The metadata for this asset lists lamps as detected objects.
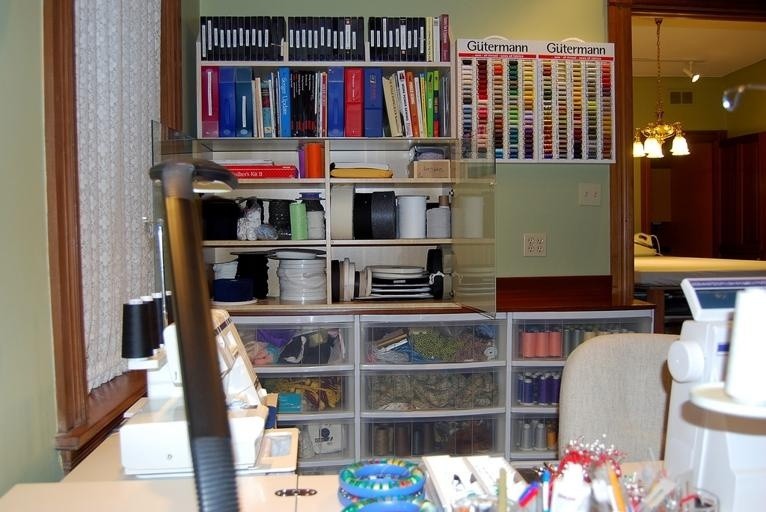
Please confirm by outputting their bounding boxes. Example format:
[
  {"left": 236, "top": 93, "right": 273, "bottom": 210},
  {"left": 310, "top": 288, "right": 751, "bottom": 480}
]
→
[
  {"left": 634, "top": 19, "right": 703, "bottom": 160},
  {"left": 147, "top": 158, "right": 242, "bottom": 508},
  {"left": 720, "top": 81, "right": 764, "bottom": 113}
]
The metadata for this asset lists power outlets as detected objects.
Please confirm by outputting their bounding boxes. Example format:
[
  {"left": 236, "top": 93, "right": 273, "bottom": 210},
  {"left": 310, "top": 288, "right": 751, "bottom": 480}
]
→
[{"left": 523, "top": 232, "right": 547, "bottom": 257}]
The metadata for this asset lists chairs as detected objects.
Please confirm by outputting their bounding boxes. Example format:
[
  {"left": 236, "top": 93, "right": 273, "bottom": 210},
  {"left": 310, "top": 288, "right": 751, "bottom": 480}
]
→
[{"left": 556, "top": 329, "right": 682, "bottom": 466}]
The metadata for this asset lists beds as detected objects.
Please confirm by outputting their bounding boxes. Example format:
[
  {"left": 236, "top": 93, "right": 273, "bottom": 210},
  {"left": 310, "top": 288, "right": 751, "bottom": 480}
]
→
[{"left": 634, "top": 232, "right": 766, "bottom": 334}]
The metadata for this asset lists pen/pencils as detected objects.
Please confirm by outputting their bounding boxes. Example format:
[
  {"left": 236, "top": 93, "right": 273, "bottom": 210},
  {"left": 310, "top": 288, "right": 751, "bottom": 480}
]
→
[
  {"left": 604, "top": 448, "right": 705, "bottom": 512},
  {"left": 510, "top": 470, "right": 550, "bottom": 512},
  {"left": 499, "top": 468, "right": 506, "bottom": 512}
]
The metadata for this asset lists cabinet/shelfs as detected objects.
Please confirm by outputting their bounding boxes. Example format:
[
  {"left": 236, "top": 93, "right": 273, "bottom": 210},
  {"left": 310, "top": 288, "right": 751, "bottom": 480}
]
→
[
  {"left": 194, "top": 27, "right": 458, "bottom": 141},
  {"left": 228, "top": 310, "right": 651, "bottom": 475},
  {"left": 639, "top": 127, "right": 765, "bottom": 260},
  {"left": 153, "top": 148, "right": 454, "bottom": 314}
]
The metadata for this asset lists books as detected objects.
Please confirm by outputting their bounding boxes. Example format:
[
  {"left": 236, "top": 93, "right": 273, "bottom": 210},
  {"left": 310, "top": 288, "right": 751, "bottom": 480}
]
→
[{"left": 200, "top": 14, "right": 451, "bottom": 136}]
